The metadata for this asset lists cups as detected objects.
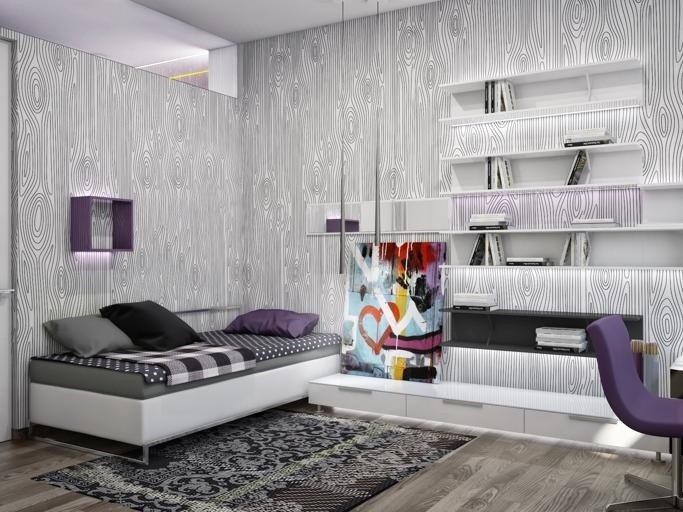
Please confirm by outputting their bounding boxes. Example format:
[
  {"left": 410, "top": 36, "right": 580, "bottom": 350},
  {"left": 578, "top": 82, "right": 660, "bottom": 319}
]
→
[{"left": 390, "top": 356, "right": 404, "bottom": 380}]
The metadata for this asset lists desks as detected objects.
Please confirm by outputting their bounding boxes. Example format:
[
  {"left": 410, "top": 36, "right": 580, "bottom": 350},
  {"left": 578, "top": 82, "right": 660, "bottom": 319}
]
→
[{"left": 665, "top": 356, "right": 683, "bottom": 474}]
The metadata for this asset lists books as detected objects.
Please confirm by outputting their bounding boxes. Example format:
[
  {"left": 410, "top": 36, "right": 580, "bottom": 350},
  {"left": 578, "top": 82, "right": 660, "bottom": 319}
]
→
[
  {"left": 571, "top": 222, "right": 619, "bottom": 229},
  {"left": 454, "top": 293, "right": 498, "bottom": 311},
  {"left": 565, "top": 150, "right": 588, "bottom": 185},
  {"left": 570, "top": 218, "right": 620, "bottom": 224},
  {"left": 484, "top": 80, "right": 515, "bottom": 113},
  {"left": 468, "top": 212, "right": 512, "bottom": 230},
  {"left": 562, "top": 129, "right": 610, "bottom": 147},
  {"left": 468, "top": 232, "right": 591, "bottom": 266},
  {"left": 534, "top": 327, "right": 586, "bottom": 354},
  {"left": 484, "top": 158, "right": 513, "bottom": 189}
]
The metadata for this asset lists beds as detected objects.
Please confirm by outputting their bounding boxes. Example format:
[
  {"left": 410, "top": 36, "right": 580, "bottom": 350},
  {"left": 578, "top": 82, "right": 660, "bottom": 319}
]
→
[{"left": 29, "top": 329, "right": 342, "bottom": 466}]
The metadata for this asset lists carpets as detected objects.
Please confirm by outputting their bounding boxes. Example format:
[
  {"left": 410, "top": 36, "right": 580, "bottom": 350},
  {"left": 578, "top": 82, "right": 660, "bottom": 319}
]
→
[{"left": 30, "top": 407, "right": 479, "bottom": 512}]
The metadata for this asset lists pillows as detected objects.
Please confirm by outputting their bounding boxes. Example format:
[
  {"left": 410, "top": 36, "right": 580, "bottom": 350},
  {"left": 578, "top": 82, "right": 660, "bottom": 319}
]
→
[
  {"left": 223, "top": 309, "right": 319, "bottom": 339},
  {"left": 99, "top": 300, "right": 206, "bottom": 351},
  {"left": 42, "top": 314, "right": 143, "bottom": 359}
]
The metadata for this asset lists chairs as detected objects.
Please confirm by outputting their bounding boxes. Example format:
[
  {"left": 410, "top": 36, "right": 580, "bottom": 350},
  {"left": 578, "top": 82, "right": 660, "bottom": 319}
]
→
[{"left": 586, "top": 315, "right": 683, "bottom": 511}]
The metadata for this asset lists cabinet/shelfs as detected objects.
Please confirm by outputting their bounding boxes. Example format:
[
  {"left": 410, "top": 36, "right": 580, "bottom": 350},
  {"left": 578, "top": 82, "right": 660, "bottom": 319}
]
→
[{"left": 309, "top": 374, "right": 672, "bottom": 463}]
